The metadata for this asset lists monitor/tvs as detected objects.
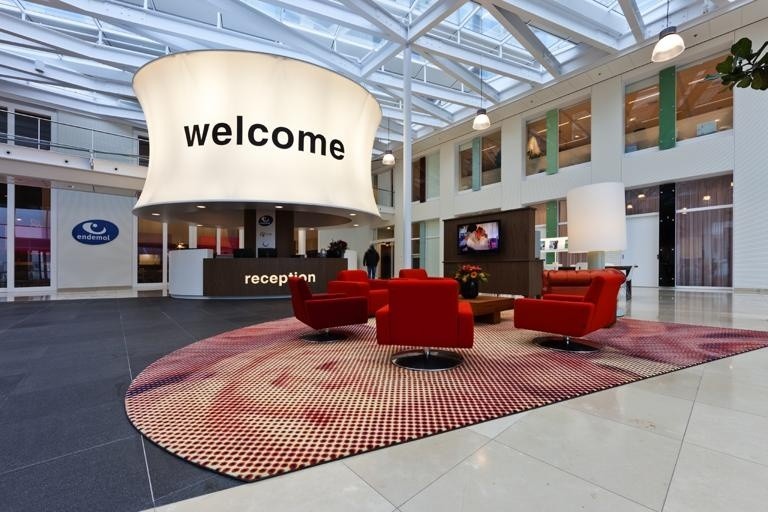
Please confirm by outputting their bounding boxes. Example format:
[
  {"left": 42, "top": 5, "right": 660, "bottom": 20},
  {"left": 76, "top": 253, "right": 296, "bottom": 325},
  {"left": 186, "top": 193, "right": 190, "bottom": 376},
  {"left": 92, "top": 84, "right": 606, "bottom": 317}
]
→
[{"left": 456, "top": 219, "right": 502, "bottom": 256}]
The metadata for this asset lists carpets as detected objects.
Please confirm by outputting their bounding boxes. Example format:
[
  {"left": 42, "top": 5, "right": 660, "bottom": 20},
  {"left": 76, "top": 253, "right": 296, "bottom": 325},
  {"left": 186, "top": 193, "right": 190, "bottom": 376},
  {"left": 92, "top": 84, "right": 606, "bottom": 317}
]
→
[{"left": 124, "top": 314, "right": 768, "bottom": 483}]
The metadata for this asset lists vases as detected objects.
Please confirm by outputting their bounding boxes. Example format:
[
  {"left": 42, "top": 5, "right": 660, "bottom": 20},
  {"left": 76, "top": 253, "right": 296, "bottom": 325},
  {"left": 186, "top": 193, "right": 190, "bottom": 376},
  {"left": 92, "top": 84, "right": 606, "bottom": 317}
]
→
[{"left": 458, "top": 277, "right": 478, "bottom": 298}]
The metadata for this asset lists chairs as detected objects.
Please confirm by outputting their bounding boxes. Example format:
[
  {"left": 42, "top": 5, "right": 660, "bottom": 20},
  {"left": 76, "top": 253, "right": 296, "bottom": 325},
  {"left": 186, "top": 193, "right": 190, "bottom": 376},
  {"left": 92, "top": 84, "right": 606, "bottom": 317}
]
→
[
  {"left": 375, "top": 278, "right": 474, "bottom": 371},
  {"left": 399, "top": 268, "right": 428, "bottom": 279},
  {"left": 287, "top": 276, "right": 369, "bottom": 344},
  {"left": 327, "top": 269, "right": 389, "bottom": 316},
  {"left": 542, "top": 269, "right": 626, "bottom": 302},
  {"left": 513, "top": 273, "right": 626, "bottom": 353}
]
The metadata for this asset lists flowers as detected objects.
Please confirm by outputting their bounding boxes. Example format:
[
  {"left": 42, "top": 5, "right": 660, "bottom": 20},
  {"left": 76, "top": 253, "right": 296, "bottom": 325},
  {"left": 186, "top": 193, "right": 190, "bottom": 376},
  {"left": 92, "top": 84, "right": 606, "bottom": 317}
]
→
[{"left": 452, "top": 263, "right": 491, "bottom": 283}]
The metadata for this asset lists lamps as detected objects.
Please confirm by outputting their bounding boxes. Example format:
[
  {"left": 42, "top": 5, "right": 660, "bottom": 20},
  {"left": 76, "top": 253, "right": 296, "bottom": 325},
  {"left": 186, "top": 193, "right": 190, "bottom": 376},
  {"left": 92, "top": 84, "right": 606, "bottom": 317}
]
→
[
  {"left": 651, "top": 0, "right": 685, "bottom": 62},
  {"left": 382, "top": 117, "right": 396, "bottom": 166},
  {"left": 472, "top": 67, "right": 490, "bottom": 131}
]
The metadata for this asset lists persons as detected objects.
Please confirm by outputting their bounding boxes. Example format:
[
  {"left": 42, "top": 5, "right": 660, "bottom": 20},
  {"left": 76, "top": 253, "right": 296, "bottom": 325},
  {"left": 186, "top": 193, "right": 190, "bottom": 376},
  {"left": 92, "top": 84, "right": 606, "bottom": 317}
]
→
[
  {"left": 465, "top": 226, "right": 489, "bottom": 250},
  {"left": 459, "top": 225, "right": 477, "bottom": 251},
  {"left": 363, "top": 244, "right": 379, "bottom": 279}
]
